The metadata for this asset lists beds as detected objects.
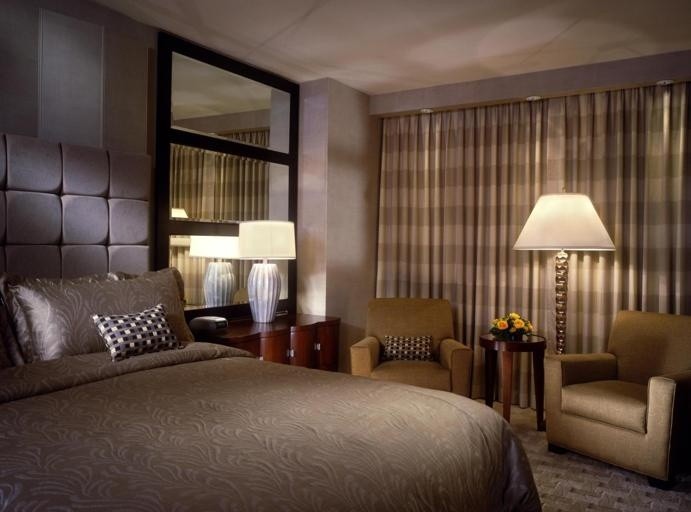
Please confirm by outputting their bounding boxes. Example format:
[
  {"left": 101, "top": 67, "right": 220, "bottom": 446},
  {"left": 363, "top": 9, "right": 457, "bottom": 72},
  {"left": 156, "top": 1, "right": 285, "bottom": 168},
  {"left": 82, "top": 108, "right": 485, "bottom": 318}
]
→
[{"left": 1, "top": 273, "right": 522, "bottom": 510}]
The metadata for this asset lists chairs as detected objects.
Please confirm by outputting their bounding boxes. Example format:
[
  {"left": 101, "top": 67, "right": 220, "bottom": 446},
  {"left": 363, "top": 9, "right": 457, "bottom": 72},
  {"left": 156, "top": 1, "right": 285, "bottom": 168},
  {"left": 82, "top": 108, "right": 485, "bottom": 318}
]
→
[
  {"left": 349, "top": 297, "right": 473, "bottom": 400},
  {"left": 544, "top": 310, "right": 690, "bottom": 491}
]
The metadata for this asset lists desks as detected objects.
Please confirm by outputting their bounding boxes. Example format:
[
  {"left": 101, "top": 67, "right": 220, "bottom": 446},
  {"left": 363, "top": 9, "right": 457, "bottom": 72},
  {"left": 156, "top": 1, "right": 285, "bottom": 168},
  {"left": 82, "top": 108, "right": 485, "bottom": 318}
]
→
[{"left": 478, "top": 335, "right": 546, "bottom": 432}]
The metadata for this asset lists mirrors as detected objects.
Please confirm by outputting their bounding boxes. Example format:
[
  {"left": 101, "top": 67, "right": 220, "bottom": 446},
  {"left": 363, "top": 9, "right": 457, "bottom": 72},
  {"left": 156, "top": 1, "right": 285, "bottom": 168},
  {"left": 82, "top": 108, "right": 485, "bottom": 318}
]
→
[{"left": 151, "top": 32, "right": 301, "bottom": 324}]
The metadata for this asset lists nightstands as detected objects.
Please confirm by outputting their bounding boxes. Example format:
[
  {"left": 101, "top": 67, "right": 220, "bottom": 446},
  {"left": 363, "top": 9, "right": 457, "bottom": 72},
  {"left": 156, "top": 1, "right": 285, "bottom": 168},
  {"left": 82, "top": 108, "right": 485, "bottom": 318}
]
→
[{"left": 216, "top": 312, "right": 341, "bottom": 376}]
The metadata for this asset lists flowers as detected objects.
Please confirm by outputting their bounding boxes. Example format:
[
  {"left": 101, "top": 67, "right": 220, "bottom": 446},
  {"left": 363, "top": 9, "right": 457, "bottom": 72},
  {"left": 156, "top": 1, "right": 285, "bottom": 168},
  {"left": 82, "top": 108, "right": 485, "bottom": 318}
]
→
[{"left": 488, "top": 311, "right": 535, "bottom": 336}]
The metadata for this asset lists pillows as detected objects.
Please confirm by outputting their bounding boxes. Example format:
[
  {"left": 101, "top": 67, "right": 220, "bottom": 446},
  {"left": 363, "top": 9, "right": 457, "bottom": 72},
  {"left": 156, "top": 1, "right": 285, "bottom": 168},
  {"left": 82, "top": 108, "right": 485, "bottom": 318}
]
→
[
  {"left": 0, "top": 266, "right": 206, "bottom": 364},
  {"left": 383, "top": 334, "right": 435, "bottom": 364}
]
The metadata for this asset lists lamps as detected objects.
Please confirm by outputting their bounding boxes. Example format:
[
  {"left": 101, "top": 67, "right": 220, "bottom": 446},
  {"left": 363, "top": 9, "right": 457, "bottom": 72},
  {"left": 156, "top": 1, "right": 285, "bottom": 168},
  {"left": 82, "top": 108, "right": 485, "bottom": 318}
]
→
[
  {"left": 239, "top": 219, "right": 298, "bottom": 324},
  {"left": 187, "top": 219, "right": 243, "bottom": 307},
  {"left": 513, "top": 190, "right": 617, "bottom": 353}
]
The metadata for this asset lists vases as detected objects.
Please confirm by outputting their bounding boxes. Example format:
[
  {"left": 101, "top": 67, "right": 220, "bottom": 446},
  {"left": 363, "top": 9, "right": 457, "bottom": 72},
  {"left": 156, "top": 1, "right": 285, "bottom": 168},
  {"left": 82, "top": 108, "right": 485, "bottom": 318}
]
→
[{"left": 503, "top": 331, "right": 522, "bottom": 342}]
